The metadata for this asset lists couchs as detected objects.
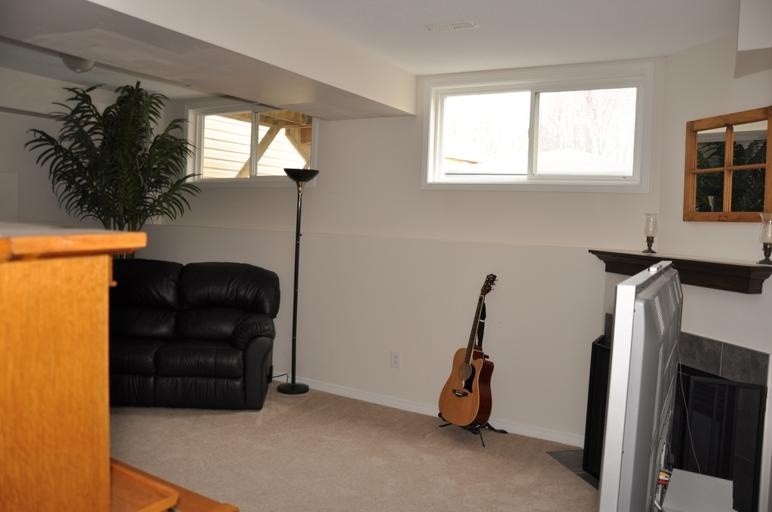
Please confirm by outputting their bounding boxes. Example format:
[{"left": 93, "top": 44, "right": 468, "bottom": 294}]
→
[{"left": 109, "top": 258, "right": 280, "bottom": 409}]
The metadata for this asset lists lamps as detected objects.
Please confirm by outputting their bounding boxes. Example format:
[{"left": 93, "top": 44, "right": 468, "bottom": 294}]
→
[{"left": 277, "top": 168, "right": 319, "bottom": 394}]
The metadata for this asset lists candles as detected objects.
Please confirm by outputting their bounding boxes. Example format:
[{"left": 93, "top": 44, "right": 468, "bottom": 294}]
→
[
  {"left": 646, "top": 215, "right": 655, "bottom": 237},
  {"left": 764, "top": 220, "right": 772, "bottom": 243}
]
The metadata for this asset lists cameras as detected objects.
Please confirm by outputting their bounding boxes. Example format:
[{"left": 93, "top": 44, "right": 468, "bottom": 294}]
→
[{"left": 600, "top": 260, "right": 735, "bottom": 512}]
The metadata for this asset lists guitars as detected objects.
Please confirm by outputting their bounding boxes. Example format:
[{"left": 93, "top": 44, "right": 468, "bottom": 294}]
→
[{"left": 438, "top": 274, "right": 498, "bottom": 426}]
